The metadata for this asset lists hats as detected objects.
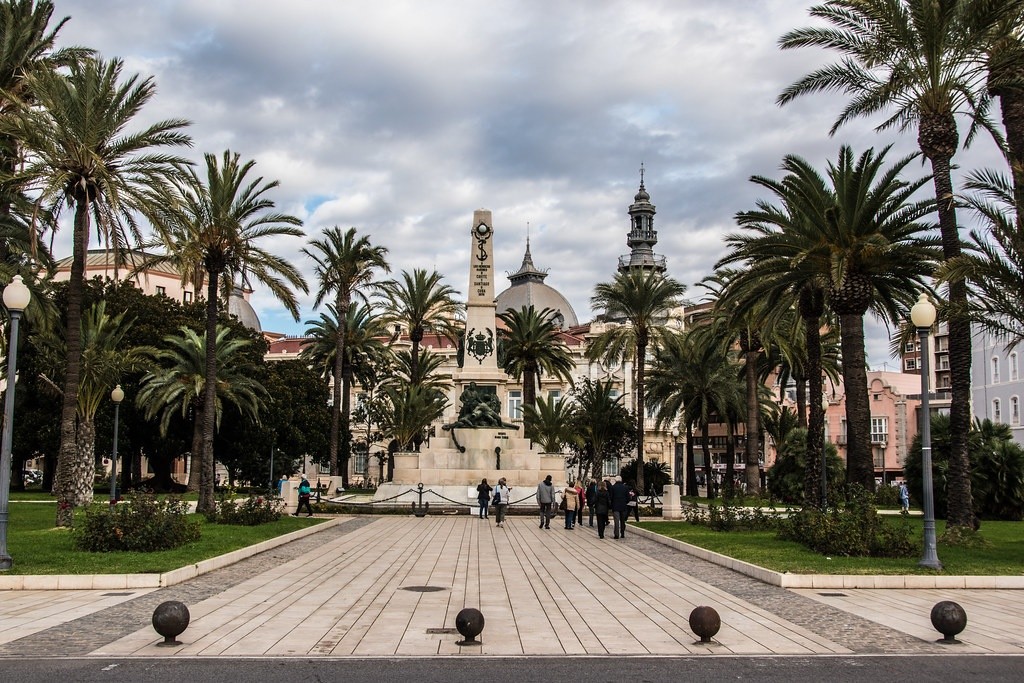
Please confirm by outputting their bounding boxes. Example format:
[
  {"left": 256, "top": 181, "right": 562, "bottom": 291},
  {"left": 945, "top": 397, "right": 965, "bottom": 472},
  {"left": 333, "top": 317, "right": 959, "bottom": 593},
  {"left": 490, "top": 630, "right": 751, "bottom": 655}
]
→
[{"left": 301, "top": 474, "right": 307, "bottom": 479}]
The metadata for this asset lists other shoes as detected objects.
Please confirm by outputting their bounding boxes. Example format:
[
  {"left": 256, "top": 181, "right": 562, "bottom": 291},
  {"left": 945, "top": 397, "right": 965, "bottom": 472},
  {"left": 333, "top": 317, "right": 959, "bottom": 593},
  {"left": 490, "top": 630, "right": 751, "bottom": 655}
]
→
[
  {"left": 621, "top": 535, "right": 625, "bottom": 538},
  {"left": 564, "top": 527, "right": 573, "bottom": 530},
  {"left": 545, "top": 525, "right": 550, "bottom": 529},
  {"left": 614, "top": 535, "right": 619, "bottom": 539},
  {"left": 479, "top": 516, "right": 484, "bottom": 519},
  {"left": 306, "top": 514, "right": 313, "bottom": 518},
  {"left": 291, "top": 513, "right": 298, "bottom": 517},
  {"left": 500, "top": 521, "right": 504, "bottom": 528},
  {"left": 485, "top": 516, "right": 489, "bottom": 519},
  {"left": 599, "top": 535, "right": 604, "bottom": 539},
  {"left": 539, "top": 521, "right": 544, "bottom": 528},
  {"left": 496, "top": 522, "right": 499, "bottom": 527},
  {"left": 901, "top": 511, "right": 909, "bottom": 514}
]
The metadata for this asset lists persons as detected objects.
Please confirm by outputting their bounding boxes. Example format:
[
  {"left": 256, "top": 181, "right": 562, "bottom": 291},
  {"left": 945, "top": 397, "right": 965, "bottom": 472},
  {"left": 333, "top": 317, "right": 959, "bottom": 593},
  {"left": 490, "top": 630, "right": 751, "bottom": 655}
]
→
[
  {"left": 443, "top": 382, "right": 504, "bottom": 431},
  {"left": 560, "top": 476, "right": 639, "bottom": 539},
  {"left": 900, "top": 481, "right": 909, "bottom": 513},
  {"left": 477, "top": 478, "right": 493, "bottom": 519},
  {"left": 491, "top": 477, "right": 510, "bottom": 528},
  {"left": 292, "top": 474, "right": 314, "bottom": 517},
  {"left": 536, "top": 475, "right": 555, "bottom": 529}
]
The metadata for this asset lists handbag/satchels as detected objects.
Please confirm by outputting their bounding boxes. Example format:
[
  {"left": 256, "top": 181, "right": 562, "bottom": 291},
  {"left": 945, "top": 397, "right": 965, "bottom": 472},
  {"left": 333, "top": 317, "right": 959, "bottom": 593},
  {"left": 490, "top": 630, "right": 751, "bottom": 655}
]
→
[
  {"left": 559, "top": 489, "right": 567, "bottom": 510},
  {"left": 491, "top": 492, "right": 501, "bottom": 505},
  {"left": 897, "top": 497, "right": 903, "bottom": 505},
  {"left": 487, "top": 496, "right": 490, "bottom": 501}
]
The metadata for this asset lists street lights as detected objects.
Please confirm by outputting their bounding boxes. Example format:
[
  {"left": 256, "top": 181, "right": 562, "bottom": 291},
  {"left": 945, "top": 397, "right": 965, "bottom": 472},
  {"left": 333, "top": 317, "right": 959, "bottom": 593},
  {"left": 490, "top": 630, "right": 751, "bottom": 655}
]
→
[
  {"left": 910, "top": 292, "right": 942, "bottom": 570},
  {"left": 672, "top": 428, "right": 680, "bottom": 484},
  {"left": 0, "top": 275, "right": 31, "bottom": 570},
  {"left": 109, "top": 385, "right": 125, "bottom": 511}
]
